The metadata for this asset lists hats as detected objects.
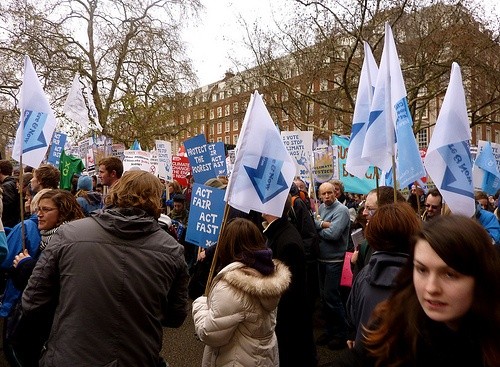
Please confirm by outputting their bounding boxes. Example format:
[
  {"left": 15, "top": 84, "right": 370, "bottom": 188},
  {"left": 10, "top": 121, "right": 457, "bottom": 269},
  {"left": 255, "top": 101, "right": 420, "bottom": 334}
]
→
[{"left": 78, "top": 176, "right": 92, "bottom": 190}]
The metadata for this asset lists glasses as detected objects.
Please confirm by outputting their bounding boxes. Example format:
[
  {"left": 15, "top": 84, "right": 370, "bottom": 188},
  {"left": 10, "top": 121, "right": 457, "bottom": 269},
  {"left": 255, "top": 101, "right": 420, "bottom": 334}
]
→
[
  {"left": 424, "top": 202, "right": 442, "bottom": 210},
  {"left": 35, "top": 207, "right": 58, "bottom": 215}
]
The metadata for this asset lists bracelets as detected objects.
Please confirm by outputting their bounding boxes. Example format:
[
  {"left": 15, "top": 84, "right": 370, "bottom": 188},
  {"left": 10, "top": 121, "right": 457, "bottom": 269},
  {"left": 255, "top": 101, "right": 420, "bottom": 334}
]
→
[{"left": 320, "top": 221, "right": 324, "bottom": 228}]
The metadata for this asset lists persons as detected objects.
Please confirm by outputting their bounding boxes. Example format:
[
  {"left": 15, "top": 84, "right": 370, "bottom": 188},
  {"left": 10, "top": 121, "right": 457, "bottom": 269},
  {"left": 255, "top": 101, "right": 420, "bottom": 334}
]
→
[
  {"left": 0, "top": 157, "right": 500, "bottom": 367},
  {"left": 190, "top": 218, "right": 293, "bottom": 367},
  {"left": 21, "top": 170, "right": 190, "bottom": 367}
]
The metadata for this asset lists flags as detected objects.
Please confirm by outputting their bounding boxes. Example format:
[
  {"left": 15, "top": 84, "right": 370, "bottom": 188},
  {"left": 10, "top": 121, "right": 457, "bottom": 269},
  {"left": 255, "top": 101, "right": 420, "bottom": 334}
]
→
[{"left": 11, "top": 23, "right": 500, "bottom": 219}]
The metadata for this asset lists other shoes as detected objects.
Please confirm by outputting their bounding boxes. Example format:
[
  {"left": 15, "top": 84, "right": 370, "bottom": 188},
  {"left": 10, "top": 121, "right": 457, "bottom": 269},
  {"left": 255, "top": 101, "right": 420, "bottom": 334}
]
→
[
  {"left": 328, "top": 340, "right": 344, "bottom": 350},
  {"left": 316, "top": 333, "right": 334, "bottom": 346}
]
